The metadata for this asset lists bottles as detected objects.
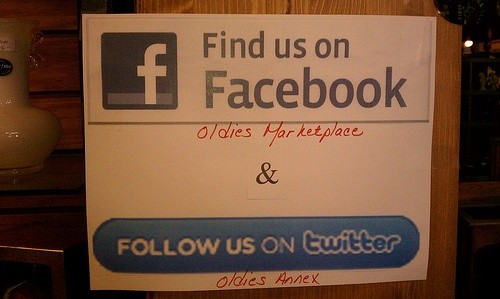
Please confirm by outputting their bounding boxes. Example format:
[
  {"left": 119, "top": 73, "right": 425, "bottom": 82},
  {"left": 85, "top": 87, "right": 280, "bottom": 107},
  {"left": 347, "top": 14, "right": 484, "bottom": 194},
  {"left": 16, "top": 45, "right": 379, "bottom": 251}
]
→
[{"left": 0, "top": 18, "right": 62, "bottom": 177}]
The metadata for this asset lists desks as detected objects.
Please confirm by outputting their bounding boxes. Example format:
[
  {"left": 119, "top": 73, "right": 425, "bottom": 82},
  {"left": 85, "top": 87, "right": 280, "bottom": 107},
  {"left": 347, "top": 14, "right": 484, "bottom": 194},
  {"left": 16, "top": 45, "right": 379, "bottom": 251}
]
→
[{"left": 0, "top": 162, "right": 89, "bottom": 298}]
